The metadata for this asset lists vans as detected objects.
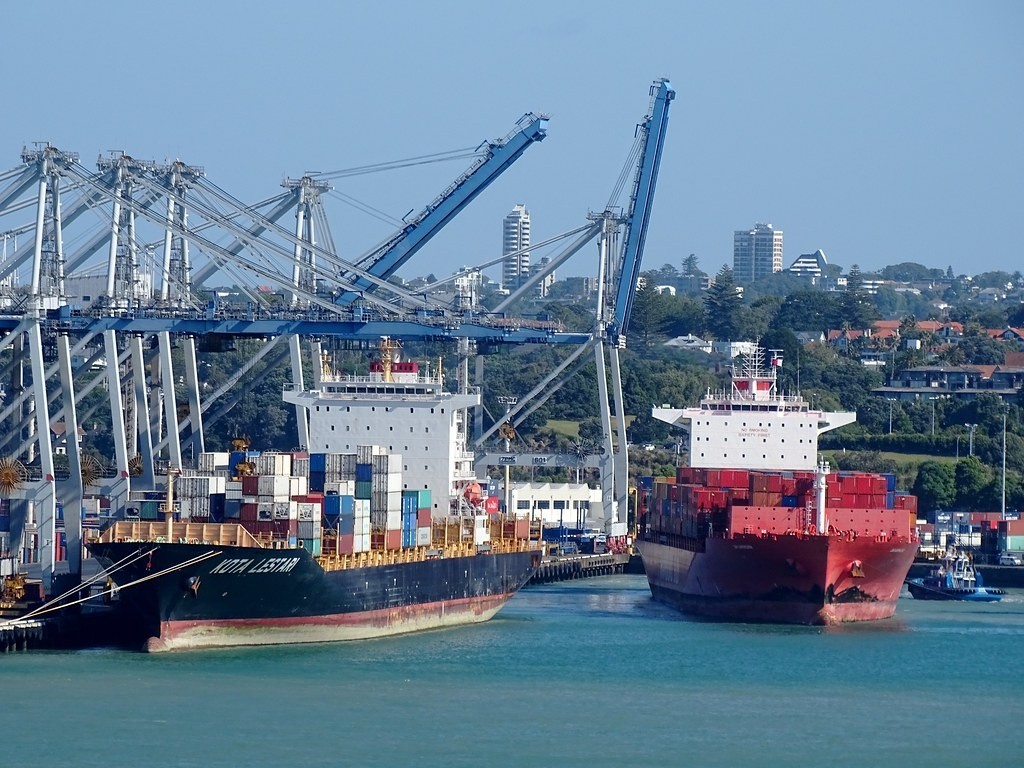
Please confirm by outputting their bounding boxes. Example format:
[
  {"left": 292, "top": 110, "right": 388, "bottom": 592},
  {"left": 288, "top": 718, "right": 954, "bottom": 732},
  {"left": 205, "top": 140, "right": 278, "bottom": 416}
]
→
[{"left": 1000, "top": 555, "right": 1022, "bottom": 566}]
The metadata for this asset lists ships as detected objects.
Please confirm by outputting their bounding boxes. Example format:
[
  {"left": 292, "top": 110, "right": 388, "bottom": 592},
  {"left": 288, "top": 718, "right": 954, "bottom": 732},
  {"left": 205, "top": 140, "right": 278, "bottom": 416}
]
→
[
  {"left": 631, "top": 336, "right": 920, "bottom": 626},
  {"left": 83, "top": 334, "right": 545, "bottom": 654}
]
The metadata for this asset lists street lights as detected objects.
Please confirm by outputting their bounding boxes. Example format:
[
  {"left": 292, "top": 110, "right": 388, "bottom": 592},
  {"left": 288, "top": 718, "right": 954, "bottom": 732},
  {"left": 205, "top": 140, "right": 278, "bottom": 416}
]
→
[
  {"left": 887, "top": 398, "right": 897, "bottom": 434},
  {"left": 929, "top": 396, "right": 940, "bottom": 436},
  {"left": 965, "top": 423, "right": 977, "bottom": 456}
]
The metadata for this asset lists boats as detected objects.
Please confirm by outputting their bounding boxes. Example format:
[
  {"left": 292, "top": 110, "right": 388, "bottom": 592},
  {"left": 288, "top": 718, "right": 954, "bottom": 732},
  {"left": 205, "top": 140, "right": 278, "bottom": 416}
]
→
[{"left": 906, "top": 550, "right": 1005, "bottom": 602}]
